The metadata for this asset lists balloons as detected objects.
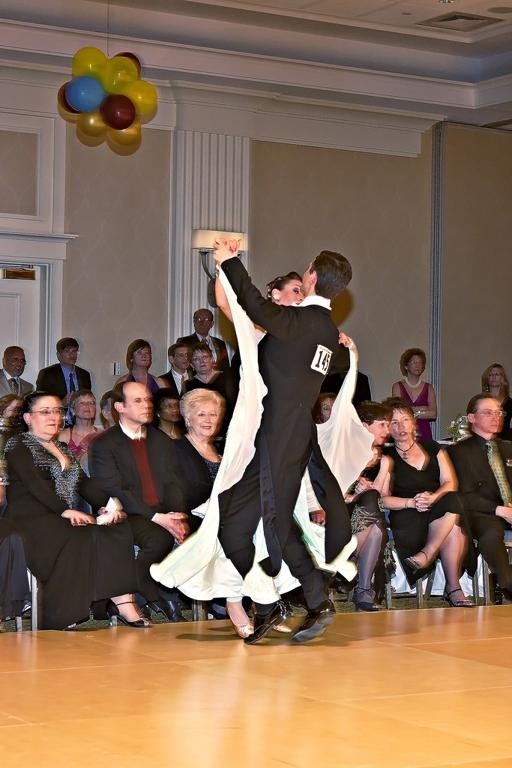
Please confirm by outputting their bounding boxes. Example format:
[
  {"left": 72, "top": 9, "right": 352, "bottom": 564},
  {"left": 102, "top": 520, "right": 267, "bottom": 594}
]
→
[{"left": 56, "top": 46, "right": 159, "bottom": 156}]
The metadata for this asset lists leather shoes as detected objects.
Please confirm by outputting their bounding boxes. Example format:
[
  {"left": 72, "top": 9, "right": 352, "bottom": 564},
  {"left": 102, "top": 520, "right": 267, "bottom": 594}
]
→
[
  {"left": 227, "top": 599, "right": 336, "bottom": 645},
  {"left": 143, "top": 598, "right": 188, "bottom": 622},
  {"left": 489, "top": 573, "right": 502, "bottom": 605}
]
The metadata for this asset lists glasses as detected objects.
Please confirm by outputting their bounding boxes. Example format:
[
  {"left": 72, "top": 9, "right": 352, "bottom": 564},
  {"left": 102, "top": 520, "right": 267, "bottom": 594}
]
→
[{"left": 25, "top": 408, "right": 61, "bottom": 417}]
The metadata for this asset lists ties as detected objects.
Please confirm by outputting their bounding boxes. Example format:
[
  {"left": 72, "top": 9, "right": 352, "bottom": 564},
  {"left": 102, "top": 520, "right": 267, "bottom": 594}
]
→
[
  {"left": 202, "top": 337, "right": 207, "bottom": 344},
  {"left": 10, "top": 379, "right": 15, "bottom": 392},
  {"left": 487, "top": 440, "right": 512, "bottom": 508},
  {"left": 70, "top": 374, "right": 76, "bottom": 420},
  {"left": 181, "top": 376, "right": 185, "bottom": 393}
]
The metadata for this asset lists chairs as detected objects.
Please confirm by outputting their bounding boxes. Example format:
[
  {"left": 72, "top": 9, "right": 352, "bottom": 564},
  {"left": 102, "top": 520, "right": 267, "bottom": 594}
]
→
[{"left": 1, "top": 512, "right": 512, "bottom": 630}]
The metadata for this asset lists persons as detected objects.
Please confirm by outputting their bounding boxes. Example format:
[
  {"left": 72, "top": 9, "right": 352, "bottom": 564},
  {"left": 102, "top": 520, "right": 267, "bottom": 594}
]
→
[{"left": 0, "top": 308, "right": 512, "bottom": 632}]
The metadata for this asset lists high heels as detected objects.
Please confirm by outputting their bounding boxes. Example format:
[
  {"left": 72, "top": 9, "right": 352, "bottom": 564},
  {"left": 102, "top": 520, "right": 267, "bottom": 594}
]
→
[
  {"left": 406, "top": 551, "right": 429, "bottom": 574},
  {"left": 352, "top": 587, "right": 379, "bottom": 612},
  {"left": 443, "top": 588, "right": 475, "bottom": 607},
  {"left": 106, "top": 598, "right": 152, "bottom": 628}
]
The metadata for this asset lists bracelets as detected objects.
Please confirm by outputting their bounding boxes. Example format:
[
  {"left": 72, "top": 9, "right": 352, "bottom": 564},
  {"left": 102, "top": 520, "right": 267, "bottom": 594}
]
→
[{"left": 214, "top": 269, "right": 219, "bottom": 277}]
